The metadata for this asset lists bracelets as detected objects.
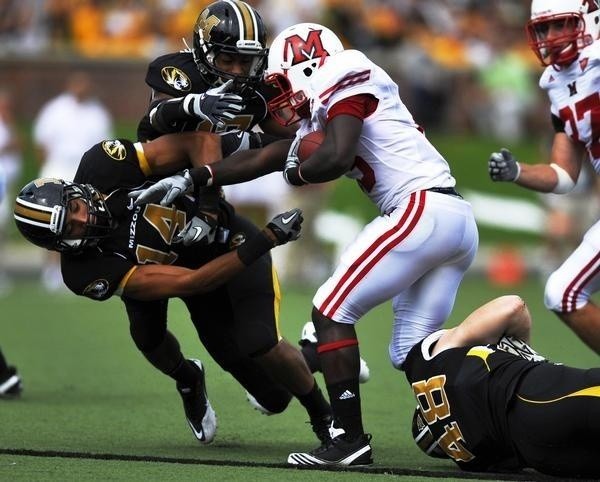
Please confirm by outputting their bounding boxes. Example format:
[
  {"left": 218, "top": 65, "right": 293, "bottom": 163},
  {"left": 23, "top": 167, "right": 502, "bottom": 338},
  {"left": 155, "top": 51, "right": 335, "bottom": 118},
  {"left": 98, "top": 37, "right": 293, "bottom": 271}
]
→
[
  {"left": 192, "top": 162, "right": 213, "bottom": 189},
  {"left": 237, "top": 230, "right": 276, "bottom": 266},
  {"left": 198, "top": 186, "right": 224, "bottom": 214},
  {"left": 283, "top": 164, "right": 311, "bottom": 188}
]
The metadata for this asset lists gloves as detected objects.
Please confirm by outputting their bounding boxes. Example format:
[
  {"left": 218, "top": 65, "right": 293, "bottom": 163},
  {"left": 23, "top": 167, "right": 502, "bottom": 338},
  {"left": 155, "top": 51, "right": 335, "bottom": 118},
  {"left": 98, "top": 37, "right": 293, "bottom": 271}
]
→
[
  {"left": 127, "top": 167, "right": 196, "bottom": 208},
  {"left": 170, "top": 215, "right": 218, "bottom": 250},
  {"left": 265, "top": 207, "right": 305, "bottom": 246},
  {"left": 488, "top": 147, "right": 521, "bottom": 184},
  {"left": 183, "top": 79, "right": 244, "bottom": 130},
  {"left": 282, "top": 134, "right": 306, "bottom": 189}
]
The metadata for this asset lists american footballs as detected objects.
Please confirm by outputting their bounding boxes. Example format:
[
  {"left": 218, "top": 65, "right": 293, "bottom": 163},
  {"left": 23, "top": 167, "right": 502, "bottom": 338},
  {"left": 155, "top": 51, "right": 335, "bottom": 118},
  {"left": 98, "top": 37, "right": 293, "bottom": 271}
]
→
[{"left": 294, "top": 128, "right": 327, "bottom": 163}]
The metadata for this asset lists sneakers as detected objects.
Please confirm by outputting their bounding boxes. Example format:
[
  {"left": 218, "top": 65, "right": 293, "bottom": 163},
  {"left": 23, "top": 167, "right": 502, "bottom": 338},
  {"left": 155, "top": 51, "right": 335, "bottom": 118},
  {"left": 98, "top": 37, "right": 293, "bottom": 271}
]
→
[
  {"left": 497, "top": 334, "right": 547, "bottom": 366},
  {"left": 176, "top": 359, "right": 219, "bottom": 446},
  {"left": 1, "top": 366, "right": 22, "bottom": 398},
  {"left": 298, "top": 320, "right": 370, "bottom": 384},
  {"left": 286, "top": 419, "right": 375, "bottom": 468},
  {"left": 243, "top": 390, "right": 273, "bottom": 416},
  {"left": 304, "top": 413, "right": 334, "bottom": 445}
]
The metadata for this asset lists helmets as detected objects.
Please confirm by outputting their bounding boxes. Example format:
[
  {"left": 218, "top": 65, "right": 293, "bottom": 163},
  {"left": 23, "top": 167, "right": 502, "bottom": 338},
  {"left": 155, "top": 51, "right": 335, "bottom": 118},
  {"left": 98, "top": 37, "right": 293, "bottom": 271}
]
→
[
  {"left": 525, "top": 0, "right": 600, "bottom": 73},
  {"left": 192, "top": 0, "right": 269, "bottom": 103},
  {"left": 263, "top": 22, "right": 345, "bottom": 127},
  {"left": 12, "top": 178, "right": 117, "bottom": 255},
  {"left": 411, "top": 405, "right": 453, "bottom": 460}
]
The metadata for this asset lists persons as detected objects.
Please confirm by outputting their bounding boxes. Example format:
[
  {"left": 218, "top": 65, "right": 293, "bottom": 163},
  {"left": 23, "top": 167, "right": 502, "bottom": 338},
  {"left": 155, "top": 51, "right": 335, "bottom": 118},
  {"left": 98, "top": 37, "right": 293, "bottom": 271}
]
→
[
  {"left": 30, "top": 70, "right": 112, "bottom": 291},
  {"left": 0, "top": 90, "right": 23, "bottom": 254},
  {"left": 1, "top": 352, "right": 19, "bottom": 395},
  {"left": 487, "top": 1, "right": 600, "bottom": 356},
  {"left": 126, "top": 2, "right": 282, "bottom": 445},
  {"left": 405, "top": 296, "right": 600, "bottom": 479},
  {"left": 267, "top": 23, "right": 481, "bottom": 469},
  {"left": 14, "top": 133, "right": 369, "bottom": 446}
]
